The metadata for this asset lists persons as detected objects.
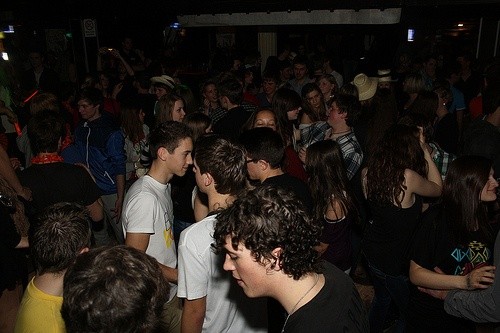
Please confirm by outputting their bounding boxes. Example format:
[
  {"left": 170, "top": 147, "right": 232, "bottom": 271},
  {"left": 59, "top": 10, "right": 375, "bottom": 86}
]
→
[{"left": 0, "top": 42, "right": 500, "bottom": 333}]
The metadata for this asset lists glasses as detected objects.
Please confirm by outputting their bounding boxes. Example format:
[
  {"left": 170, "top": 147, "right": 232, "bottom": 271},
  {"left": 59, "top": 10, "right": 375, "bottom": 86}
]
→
[{"left": 245, "top": 159, "right": 257, "bottom": 163}]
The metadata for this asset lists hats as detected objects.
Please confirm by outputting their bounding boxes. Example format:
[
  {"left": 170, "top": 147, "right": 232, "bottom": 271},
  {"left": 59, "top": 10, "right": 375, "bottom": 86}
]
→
[
  {"left": 149, "top": 74, "right": 175, "bottom": 90},
  {"left": 350, "top": 73, "right": 378, "bottom": 101},
  {"left": 376, "top": 69, "right": 398, "bottom": 82}
]
